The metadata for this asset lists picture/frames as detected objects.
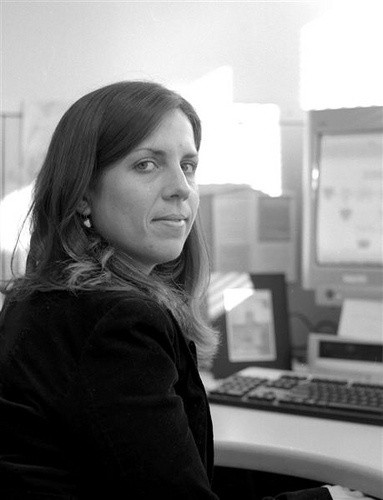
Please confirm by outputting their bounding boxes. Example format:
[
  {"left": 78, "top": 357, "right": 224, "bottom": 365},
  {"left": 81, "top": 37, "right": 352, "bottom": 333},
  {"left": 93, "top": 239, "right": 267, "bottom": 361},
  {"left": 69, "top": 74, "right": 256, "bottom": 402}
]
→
[{"left": 212, "top": 273, "right": 292, "bottom": 378}]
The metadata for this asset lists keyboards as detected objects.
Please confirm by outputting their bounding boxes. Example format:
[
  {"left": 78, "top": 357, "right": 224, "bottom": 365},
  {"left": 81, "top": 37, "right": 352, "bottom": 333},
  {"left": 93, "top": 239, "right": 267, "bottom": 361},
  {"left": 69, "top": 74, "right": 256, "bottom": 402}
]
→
[{"left": 208, "top": 367, "right": 383, "bottom": 427}]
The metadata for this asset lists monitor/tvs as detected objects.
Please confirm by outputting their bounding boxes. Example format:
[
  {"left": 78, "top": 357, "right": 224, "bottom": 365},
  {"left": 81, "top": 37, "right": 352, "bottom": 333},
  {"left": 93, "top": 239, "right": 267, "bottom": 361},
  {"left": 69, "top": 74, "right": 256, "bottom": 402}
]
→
[{"left": 303, "top": 106, "right": 383, "bottom": 300}]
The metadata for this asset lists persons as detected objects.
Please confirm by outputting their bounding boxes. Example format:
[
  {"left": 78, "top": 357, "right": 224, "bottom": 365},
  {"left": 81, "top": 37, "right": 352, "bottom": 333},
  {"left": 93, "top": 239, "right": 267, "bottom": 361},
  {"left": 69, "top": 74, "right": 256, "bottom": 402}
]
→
[{"left": 0, "top": 81, "right": 375, "bottom": 500}]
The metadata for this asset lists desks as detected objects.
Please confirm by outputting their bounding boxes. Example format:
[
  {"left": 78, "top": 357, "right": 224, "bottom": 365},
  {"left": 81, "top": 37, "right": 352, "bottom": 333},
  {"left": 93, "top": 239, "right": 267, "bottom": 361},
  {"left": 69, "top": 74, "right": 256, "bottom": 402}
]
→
[{"left": 201, "top": 398, "right": 383, "bottom": 500}]
